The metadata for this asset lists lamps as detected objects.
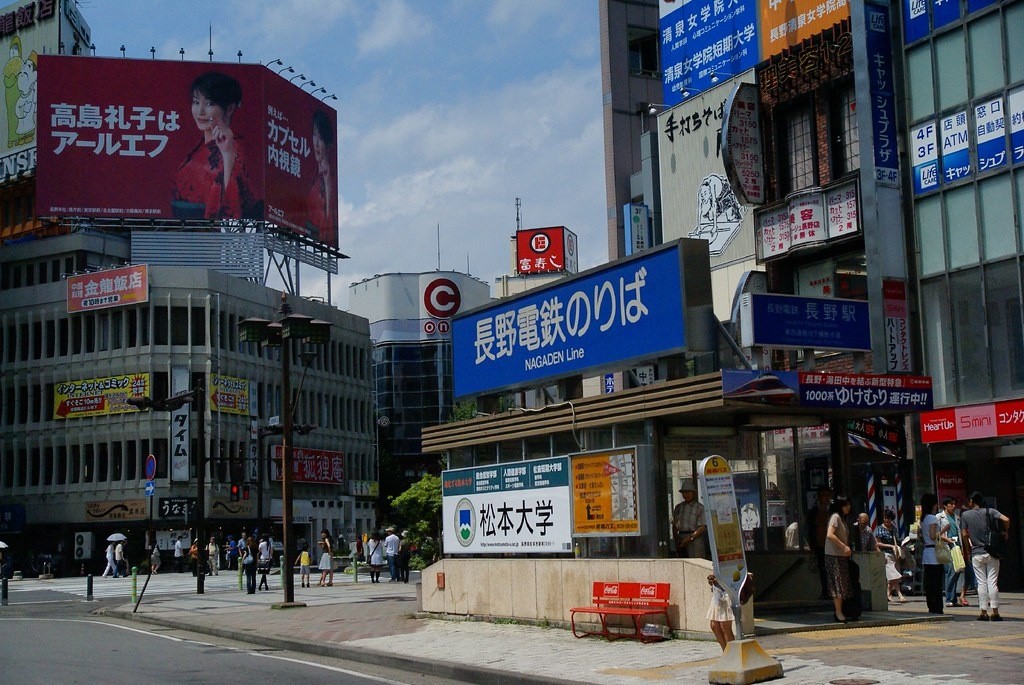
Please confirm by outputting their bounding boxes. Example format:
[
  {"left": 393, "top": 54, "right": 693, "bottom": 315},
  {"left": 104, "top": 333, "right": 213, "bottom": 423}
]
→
[
  {"left": 110, "top": 263, "right": 123, "bottom": 270},
  {"left": 85, "top": 269, "right": 96, "bottom": 273},
  {"left": 372, "top": 504, "right": 377, "bottom": 509},
  {"left": 60, "top": 273, "right": 72, "bottom": 278},
  {"left": 648, "top": 103, "right": 671, "bottom": 114},
  {"left": 278, "top": 225, "right": 340, "bottom": 254},
  {"left": 124, "top": 262, "right": 136, "bottom": 267},
  {"left": 364, "top": 502, "right": 369, "bottom": 509},
  {"left": 709, "top": 71, "right": 735, "bottom": 83},
  {"left": 680, "top": 86, "right": 703, "bottom": 101},
  {"left": 89, "top": 215, "right": 94, "bottom": 225},
  {"left": 58, "top": 215, "right": 64, "bottom": 226},
  {"left": 72, "top": 271, "right": 85, "bottom": 275},
  {"left": 57, "top": 43, "right": 337, "bottom": 103},
  {"left": 464, "top": 271, "right": 488, "bottom": 285},
  {"left": 352, "top": 273, "right": 381, "bottom": 286},
  {"left": 311, "top": 500, "right": 343, "bottom": 507},
  {"left": 356, "top": 502, "right": 361, "bottom": 508},
  {"left": 98, "top": 266, "right": 108, "bottom": 271},
  {"left": 118, "top": 216, "right": 246, "bottom": 228}
]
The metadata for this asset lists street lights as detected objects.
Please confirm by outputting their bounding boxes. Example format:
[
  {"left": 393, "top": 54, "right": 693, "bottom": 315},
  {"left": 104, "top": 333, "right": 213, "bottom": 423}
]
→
[{"left": 236, "top": 311, "right": 335, "bottom": 610}]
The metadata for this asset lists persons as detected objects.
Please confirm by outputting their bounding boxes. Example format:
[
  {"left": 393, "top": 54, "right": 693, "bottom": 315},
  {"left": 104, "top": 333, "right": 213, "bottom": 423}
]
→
[
  {"left": 189, "top": 535, "right": 239, "bottom": 577},
  {"left": 784, "top": 512, "right": 810, "bottom": 551},
  {"left": 337, "top": 534, "right": 365, "bottom": 556},
  {"left": 672, "top": 479, "right": 707, "bottom": 559},
  {"left": 853, "top": 491, "right": 1011, "bottom": 621},
  {"left": 169, "top": 536, "right": 184, "bottom": 572},
  {"left": 295, "top": 530, "right": 333, "bottom": 588},
  {"left": 825, "top": 495, "right": 852, "bottom": 622},
  {"left": 150, "top": 540, "right": 161, "bottom": 575},
  {"left": 236, "top": 532, "right": 273, "bottom": 594},
  {"left": 0, "top": 549, "right": 9, "bottom": 580},
  {"left": 297, "top": 110, "right": 338, "bottom": 249},
  {"left": 708, "top": 572, "right": 735, "bottom": 651},
  {"left": 368, "top": 526, "right": 411, "bottom": 584},
  {"left": 101, "top": 539, "right": 130, "bottom": 578},
  {"left": 171, "top": 72, "right": 261, "bottom": 219}
]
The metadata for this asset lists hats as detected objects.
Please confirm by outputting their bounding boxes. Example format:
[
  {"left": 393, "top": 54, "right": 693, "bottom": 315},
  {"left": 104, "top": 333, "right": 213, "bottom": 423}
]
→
[
  {"left": 401, "top": 530, "right": 410, "bottom": 537},
  {"left": 356, "top": 533, "right": 362, "bottom": 540},
  {"left": 227, "top": 535, "right": 232, "bottom": 538},
  {"left": 679, "top": 482, "right": 698, "bottom": 493},
  {"left": 193, "top": 538, "right": 198, "bottom": 543},
  {"left": 385, "top": 527, "right": 394, "bottom": 532},
  {"left": 338, "top": 534, "right": 343, "bottom": 538}
]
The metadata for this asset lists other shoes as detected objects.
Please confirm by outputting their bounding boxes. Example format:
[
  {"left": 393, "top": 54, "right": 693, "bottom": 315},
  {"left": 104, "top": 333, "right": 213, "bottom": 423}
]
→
[
  {"left": 113, "top": 575, "right": 128, "bottom": 578},
  {"left": 833, "top": 609, "right": 858, "bottom": 623},
  {"left": 977, "top": 613, "right": 1003, "bottom": 621},
  {"left": 946, "top": 596, "right": 969, "bottom": 606},
  {"left": 154, "top": 570, "right": 157, "bottom": 574},
  {"left": 302, "top": 583, "right": 309, "bottom": 587},
  {"left": 887, "top": 595, "right": 906, "bottom": 602}
]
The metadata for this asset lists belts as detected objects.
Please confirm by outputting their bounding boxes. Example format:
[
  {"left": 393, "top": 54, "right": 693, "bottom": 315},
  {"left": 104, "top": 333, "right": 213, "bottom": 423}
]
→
[{"left": 679, "top": 530, "right": 694, "bottom": 534}]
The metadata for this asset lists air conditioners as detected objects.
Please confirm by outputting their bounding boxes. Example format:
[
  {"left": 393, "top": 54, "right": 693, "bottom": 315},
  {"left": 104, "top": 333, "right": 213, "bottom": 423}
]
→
[
  {"left": 75, "top": 547, "right": 93, "bottom": 560},
  {"left": 75, "top": 531, "right": 95, "bottom": 547}
]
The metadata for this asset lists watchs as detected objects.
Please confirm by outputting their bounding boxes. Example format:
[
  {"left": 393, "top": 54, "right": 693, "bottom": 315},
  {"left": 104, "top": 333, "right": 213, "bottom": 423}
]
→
[{"left": 689, "top": 535, "right": 693, "bottom": 541}]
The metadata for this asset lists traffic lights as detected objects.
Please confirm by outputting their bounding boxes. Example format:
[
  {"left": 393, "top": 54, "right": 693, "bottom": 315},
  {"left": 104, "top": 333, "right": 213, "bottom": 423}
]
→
[{"left": 230, "top": 484, "right": 241, "bottom": 502}]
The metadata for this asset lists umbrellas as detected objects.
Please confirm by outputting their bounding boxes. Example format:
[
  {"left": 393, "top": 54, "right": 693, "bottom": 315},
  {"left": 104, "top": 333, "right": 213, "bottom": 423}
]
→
[
  {"left": 106, "top": 533, "right": 127, "bottom": 545},
  {"left": 0, "top": 541, "right": 8, "bottom": 548}
]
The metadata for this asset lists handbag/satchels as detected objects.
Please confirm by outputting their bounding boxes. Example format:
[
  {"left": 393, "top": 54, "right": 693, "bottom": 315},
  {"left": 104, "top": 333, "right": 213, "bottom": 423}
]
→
[
  {"left": 117, "top": 559, "right": 127, "bottom": 570},
  {"left": 257, "top": 559, "right": 270, "bottom": 574},
  {"left": 848, "top": 552, "right": 859, "bottom": 576},
  {"left": 794, "top": 537, "right": 810, "bottom": 551},
  {"left": 950, "top": 540, "right": 965, "bottom": 573},
  {"left": 243, "top": 553, "right": 254, "bottom": 565},
  {"left": 367, "top": 556, "right": 371, "bottom": 563},
  {"left": 983, "top": 529, "right": 1006, "bottom": 559},
  {"left": 935, "top": 532, "right": 952, "bottom": 563},
  {"left": 894, "top": 545, "right": 905, "bottom": 562}
]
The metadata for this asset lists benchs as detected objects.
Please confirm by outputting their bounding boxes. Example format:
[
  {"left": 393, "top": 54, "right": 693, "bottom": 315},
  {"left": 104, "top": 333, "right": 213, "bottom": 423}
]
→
[{"left": 570, "top": 581, "right": 672, "bottom": 644}]
